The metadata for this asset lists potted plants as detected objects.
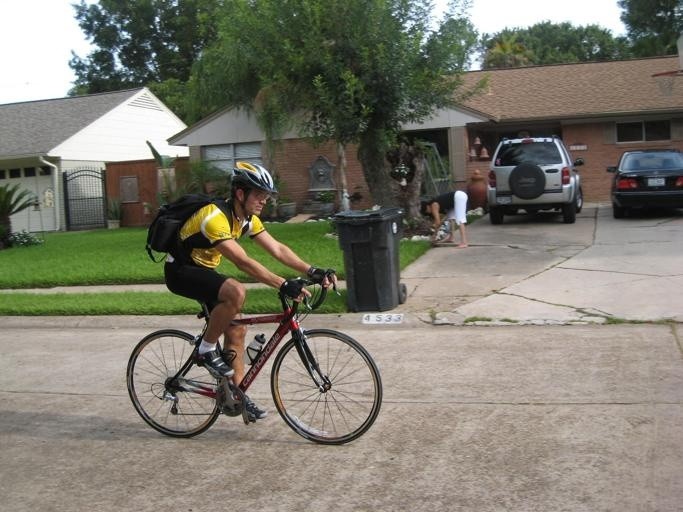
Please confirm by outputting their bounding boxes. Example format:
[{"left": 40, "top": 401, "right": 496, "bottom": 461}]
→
[
  {"left": 317, "top": 191, "right": 335, "bottom": 218},
  {"left": 102, "top": 195, "right": 125, "bottom": 230},
  {"left": 277, "top": 197, "right": 297, "bottom": 219},
  {"left": 0, "top": 182, "right": 41, "bottom": 250}
]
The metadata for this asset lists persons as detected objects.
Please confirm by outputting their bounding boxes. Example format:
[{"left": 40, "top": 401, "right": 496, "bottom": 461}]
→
[
  {"left": 419, "top": 190, "right": 469, "bottom": 249},
  {"left": 164, "top": 161, "right": 339, "bottom": 419}
]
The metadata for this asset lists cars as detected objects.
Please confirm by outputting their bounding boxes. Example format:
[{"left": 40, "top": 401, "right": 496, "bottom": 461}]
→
[{"left": 606, "top": 149, "right": 683, "bottom": 218}]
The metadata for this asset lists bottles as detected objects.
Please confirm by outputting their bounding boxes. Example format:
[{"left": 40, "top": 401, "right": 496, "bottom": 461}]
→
[{"left": 242, "top": 334, "right": 265, "bottom": 366}]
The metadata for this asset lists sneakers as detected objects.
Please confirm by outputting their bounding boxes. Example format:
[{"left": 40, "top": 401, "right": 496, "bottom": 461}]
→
[
  {"left": 243, "top": 395, "right": 267, "bottom": 418},
  {"left": 192, "top": 348, "right": 234, "bottom": 378}
]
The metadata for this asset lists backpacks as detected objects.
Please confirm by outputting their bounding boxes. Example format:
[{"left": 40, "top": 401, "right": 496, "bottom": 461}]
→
[{"left": 146, "top": 192, "right": 233, "bottom": 264}]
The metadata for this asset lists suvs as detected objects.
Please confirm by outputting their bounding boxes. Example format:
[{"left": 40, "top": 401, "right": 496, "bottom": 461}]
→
[{"left": 486, "top": 135, "right": 584, "bottom": 224}]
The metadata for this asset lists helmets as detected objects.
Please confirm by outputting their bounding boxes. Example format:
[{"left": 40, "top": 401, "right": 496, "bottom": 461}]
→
[{"left": 231, "top": 162, "right": 278, "bottom": 194}]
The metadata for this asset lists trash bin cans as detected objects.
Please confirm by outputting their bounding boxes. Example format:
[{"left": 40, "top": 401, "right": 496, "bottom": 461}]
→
[{"left": 331, "top": 206, "right": 407, "bottom": 313}]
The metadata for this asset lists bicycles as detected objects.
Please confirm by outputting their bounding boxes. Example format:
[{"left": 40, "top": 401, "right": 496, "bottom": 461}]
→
[{"left": 127, "top": 278, "right": 382, "bottom": 444}]
